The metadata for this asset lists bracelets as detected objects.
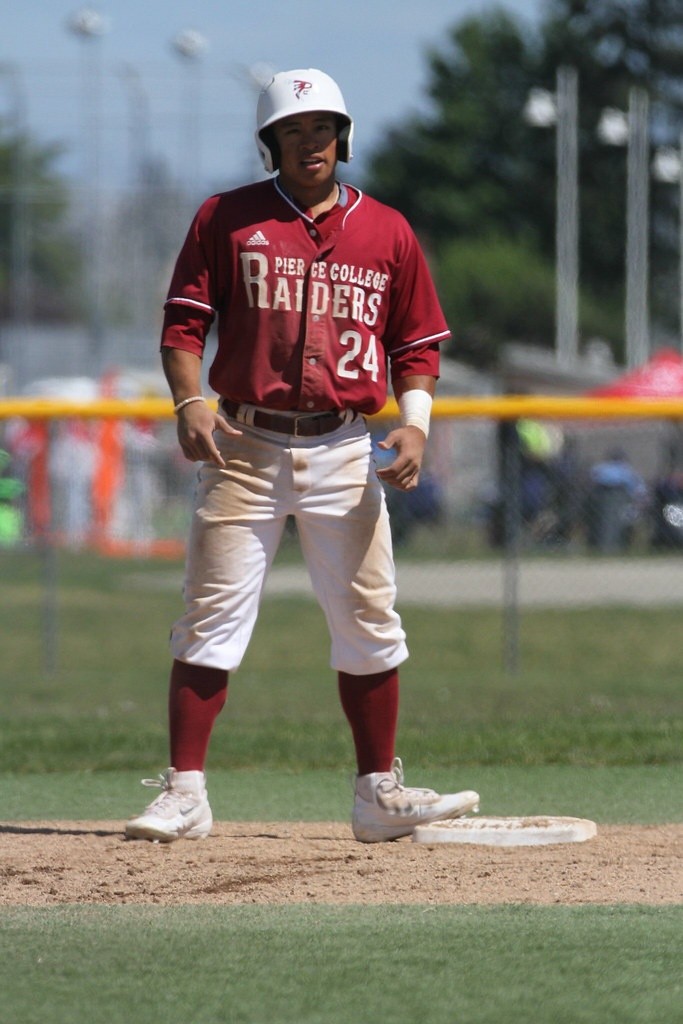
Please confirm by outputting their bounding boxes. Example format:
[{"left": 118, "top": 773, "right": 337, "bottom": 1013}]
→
[
  {"left": 174, "top": 397, "right": 206, "bottom": 414},
  {"left": 399, "top": 390, "right": 432, "bottom": 438}
]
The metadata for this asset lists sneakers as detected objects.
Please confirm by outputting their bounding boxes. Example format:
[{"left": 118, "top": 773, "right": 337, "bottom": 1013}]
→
[
  {"left": 125, "top": 768, "right": 213, "bottom": 839},
  {"left": 351, "top": 757, "right": 480, "bottom": 842}
]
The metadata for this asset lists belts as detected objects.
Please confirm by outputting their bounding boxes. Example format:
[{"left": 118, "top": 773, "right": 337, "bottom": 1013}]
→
[{"left": 222, "top": 401, "right": 357, "bottom": 436}]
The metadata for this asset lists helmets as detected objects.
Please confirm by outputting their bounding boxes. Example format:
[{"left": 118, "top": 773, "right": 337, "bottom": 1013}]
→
[{"left": 255, "top": 67, "right": 354, "bottom": 173}]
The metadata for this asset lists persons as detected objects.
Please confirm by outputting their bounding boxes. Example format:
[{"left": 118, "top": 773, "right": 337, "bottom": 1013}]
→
[
  {"left": 481, "top": 415, "right": 683, "bottom": 547},
  {"left": 124, "top": 69, "right": 480, "bottom": 842}
]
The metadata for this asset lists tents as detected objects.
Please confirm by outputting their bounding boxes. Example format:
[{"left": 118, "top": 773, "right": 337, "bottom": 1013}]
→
[{"left": 590, "top": 351, "right": 683, "bottom": 399}]
